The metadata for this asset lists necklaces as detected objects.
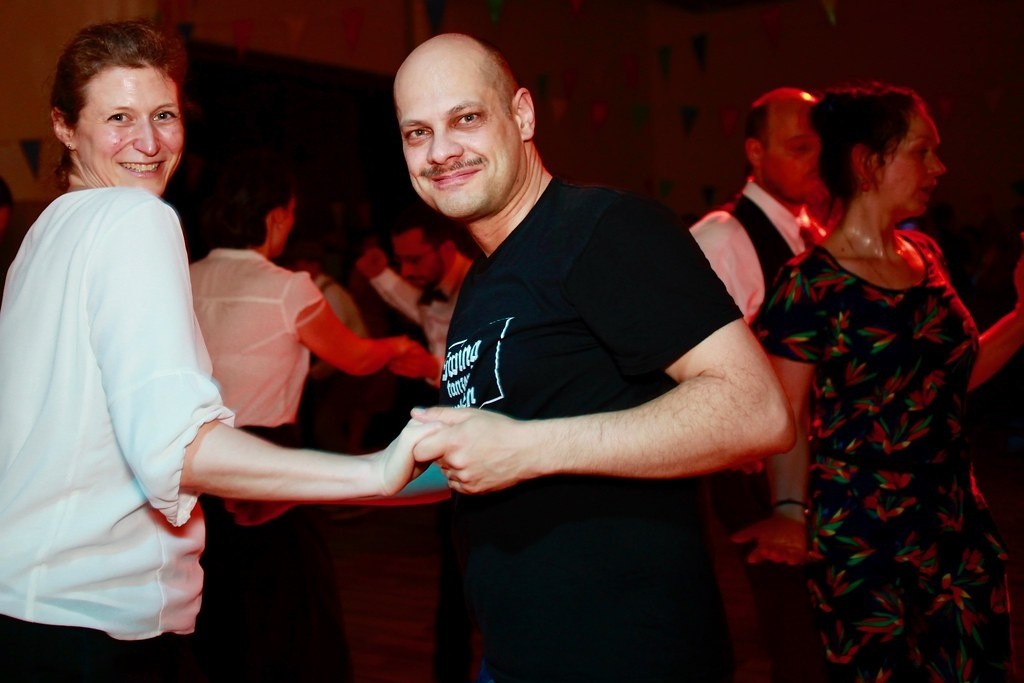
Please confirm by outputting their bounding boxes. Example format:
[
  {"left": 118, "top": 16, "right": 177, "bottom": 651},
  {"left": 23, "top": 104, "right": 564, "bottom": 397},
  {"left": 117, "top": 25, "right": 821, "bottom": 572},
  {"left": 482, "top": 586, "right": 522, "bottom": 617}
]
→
[{"left": 842, "top": 225, "right": 916, "bottom": 289}]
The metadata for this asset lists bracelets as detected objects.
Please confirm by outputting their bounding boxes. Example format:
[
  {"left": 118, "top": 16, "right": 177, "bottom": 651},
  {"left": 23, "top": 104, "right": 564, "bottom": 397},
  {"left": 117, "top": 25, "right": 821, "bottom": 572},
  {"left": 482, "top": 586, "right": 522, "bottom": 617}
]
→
[{"left": 773, "top": 499, "right": 809, "bottom": 511}]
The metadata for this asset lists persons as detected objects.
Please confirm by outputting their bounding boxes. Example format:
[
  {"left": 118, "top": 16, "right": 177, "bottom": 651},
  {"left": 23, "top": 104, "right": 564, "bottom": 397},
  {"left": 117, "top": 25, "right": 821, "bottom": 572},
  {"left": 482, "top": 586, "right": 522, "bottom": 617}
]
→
[
  {"left": 189, "top": 165, "right": 473, "bottom": 680},
  {"left": 687, "top": 87, "right": 1023, "bottom": 683},
  {"left": 395, "top": 31, "right": 800, "bottom": 683},
  {"left": 0, "top": 18, "right": 446, "bottom": 683}
]
